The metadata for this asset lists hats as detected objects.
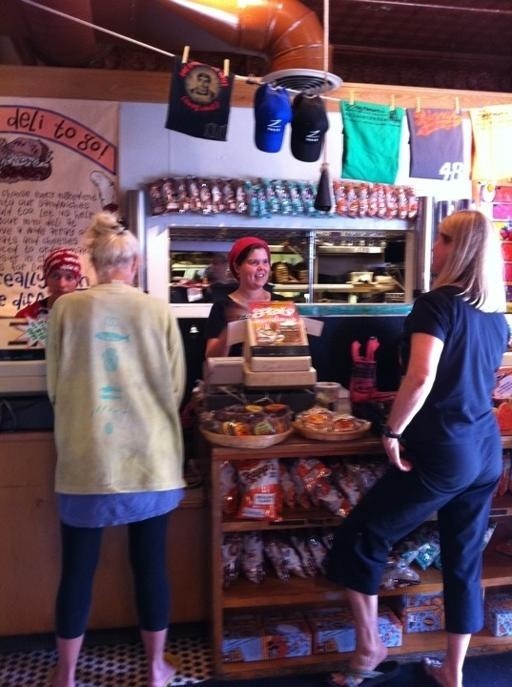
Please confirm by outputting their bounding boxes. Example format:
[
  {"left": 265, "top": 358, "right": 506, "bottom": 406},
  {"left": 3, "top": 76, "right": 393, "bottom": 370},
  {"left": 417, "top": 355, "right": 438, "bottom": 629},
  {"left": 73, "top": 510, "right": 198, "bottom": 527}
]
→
[
  {"left": 229, "top": 236, "right": 271, "bottom": 282},
  {"left": 253, "top": 83, "right": 292, "bottom": 154},
  {"left": 290, "top": 90, "right": 329, "bottom": 163}
]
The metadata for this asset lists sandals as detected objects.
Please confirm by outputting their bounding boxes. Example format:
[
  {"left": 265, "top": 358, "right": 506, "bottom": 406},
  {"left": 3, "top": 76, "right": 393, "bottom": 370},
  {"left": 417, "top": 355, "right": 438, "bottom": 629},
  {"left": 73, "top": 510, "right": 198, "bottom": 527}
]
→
[
  {"left": 160, "top": 652, "right": 179, "bottom": 686},
  {"left": 327, "top": 659, "right": 401, "bottom": 686},
  {"left": 420, "top": 656, "right": 446, "bottom": 686}
]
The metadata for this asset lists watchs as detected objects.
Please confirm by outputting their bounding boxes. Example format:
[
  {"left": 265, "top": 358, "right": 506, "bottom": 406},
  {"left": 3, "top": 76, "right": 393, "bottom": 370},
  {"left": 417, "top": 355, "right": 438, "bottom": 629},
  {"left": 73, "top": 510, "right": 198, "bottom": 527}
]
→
[{"left": 380, "top": 424, "right": 401, "bottom": 439}]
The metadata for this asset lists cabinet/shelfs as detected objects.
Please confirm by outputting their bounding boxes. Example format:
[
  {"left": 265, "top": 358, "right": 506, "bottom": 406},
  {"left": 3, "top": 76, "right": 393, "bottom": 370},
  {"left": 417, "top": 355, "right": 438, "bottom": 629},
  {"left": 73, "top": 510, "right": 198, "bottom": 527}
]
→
[{"left": 204, "top": 439, "right": 512, "bottom": 682}]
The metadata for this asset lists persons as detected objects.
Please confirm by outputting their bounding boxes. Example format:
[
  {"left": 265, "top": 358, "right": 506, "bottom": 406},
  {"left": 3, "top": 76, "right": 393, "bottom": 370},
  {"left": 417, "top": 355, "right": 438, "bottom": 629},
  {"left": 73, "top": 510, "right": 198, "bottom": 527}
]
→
[
  {"left": 17, "top": 248, "right": 83, "bottom": 318},
  {"left": 203, "top": 237, "right": 291, "bottom": 357},
  {"left": 47, "top": 214, "right": 187, "bottom": 687},
  {"left": 190, "top": 73, "right": 216, "bottom": 99},
  {"left": 327, "top": 210, "right": 512, "bottom": 687}
]
